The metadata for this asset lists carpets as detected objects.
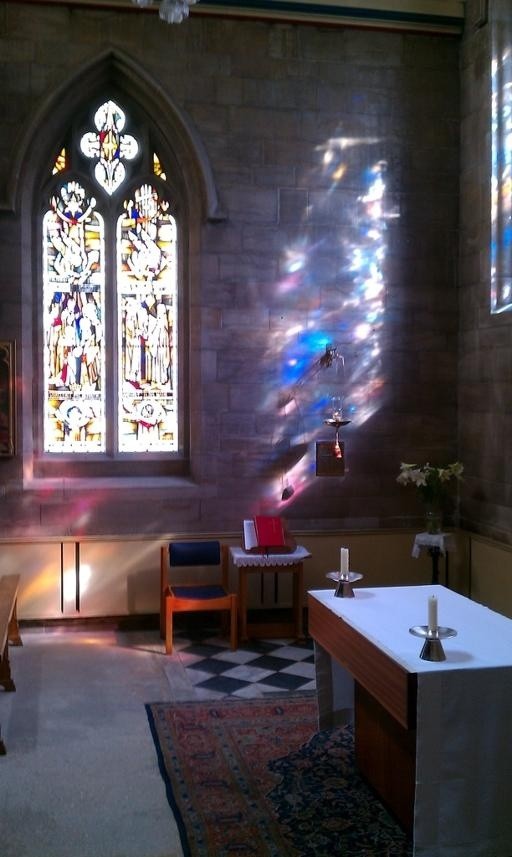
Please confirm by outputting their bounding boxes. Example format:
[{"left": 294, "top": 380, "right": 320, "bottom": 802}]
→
[{"left": 142, "top": 695, "right": 416, "bottom": 856}]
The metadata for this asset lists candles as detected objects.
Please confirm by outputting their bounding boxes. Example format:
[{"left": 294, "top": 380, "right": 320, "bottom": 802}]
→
[
  {"left": 428, "top": 593, "right": 438, "bottom": 634},
  {"left": 340, "top": 544, "right": 349, "bottom": 581}
]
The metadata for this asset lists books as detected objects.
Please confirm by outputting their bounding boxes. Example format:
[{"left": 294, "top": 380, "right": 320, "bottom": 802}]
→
[{"left": 242, "top": 514, "right": 287, "bottom": 551}]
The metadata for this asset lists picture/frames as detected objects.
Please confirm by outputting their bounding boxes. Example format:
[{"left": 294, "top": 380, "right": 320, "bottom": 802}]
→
[{"left": 1, "top": 338, "right": 17, "bottom": 459}]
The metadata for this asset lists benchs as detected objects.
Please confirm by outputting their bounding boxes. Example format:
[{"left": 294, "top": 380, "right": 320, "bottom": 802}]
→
[{"left": 1, "top": 570, "right": 28, "bottom": 757}]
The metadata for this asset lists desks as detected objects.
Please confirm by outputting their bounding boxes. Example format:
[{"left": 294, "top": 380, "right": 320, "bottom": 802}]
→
[
  {"left": 304, "top": 585, "right": 511, "bottom": 855},
  {"left": 228, "top": 543, "right": 313, "bottom": 644},
  {"left": 413, "top": 530, "right": 456, "bottom": 585}
]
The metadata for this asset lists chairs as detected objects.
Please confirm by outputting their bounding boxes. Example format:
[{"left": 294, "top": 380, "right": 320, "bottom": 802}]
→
[{"left": 157, "top": 537, "right": 241, "bottom": 656}]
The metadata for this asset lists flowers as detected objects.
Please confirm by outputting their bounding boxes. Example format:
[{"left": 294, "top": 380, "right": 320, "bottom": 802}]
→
[{"left": 395, "top": 461, "right": 467, "bottom": 502}]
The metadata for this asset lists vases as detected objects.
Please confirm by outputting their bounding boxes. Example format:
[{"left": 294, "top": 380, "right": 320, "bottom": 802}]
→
[{"left": 425, "top": 500, "right": 443, "bottom": 532}]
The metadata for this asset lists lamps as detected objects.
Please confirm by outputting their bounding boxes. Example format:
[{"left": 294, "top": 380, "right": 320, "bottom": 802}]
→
[
  {"left": 323, "top": 391, "right": 351, "bottom": 459},
  {"left": 135, "top": 1, "right": 198, "bottom": 22}
]
